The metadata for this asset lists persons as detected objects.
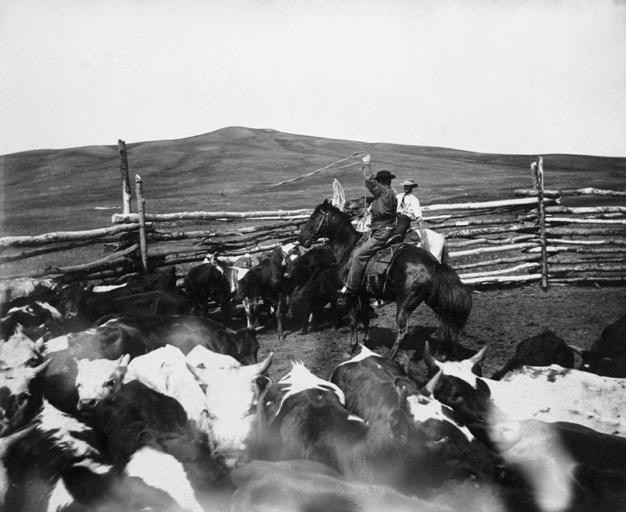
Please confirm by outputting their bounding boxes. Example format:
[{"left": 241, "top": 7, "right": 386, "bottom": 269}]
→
[
  {"left": 393, "top": 179, "right": 421, "bottom": 234},
  {"left": 342, "top": 154, "right": 397, "bottom": 295}
]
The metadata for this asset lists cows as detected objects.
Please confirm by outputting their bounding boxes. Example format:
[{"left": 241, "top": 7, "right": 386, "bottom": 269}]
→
[
  {"left": 0, "top": 310, "right": 625, "bottom": 512},
  {"left": 0, "top": 240, "right": 381, "bottom": 342}
]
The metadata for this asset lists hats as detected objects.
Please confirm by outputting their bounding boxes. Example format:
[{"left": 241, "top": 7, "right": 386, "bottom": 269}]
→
[
  {"left": 375, "top": 170, "right": 396, "bottom": 181},
  {"left": 399, "top": 179, "right": 419, "bottom": 188}
]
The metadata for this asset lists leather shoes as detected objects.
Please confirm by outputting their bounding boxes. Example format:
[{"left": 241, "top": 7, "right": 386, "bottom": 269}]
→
[{"left": 337, "top": 285, "right": 354, "bottom": 294}]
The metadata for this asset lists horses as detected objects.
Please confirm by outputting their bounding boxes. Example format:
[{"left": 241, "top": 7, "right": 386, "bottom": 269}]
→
[{"left": 297, "top": 198, "right": 472, "bottom": 360}]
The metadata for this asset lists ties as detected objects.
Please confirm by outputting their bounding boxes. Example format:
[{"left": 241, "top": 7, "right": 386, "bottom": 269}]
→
[{"left": 399, "top": 194, "right": 406, "bottom": 208}]
